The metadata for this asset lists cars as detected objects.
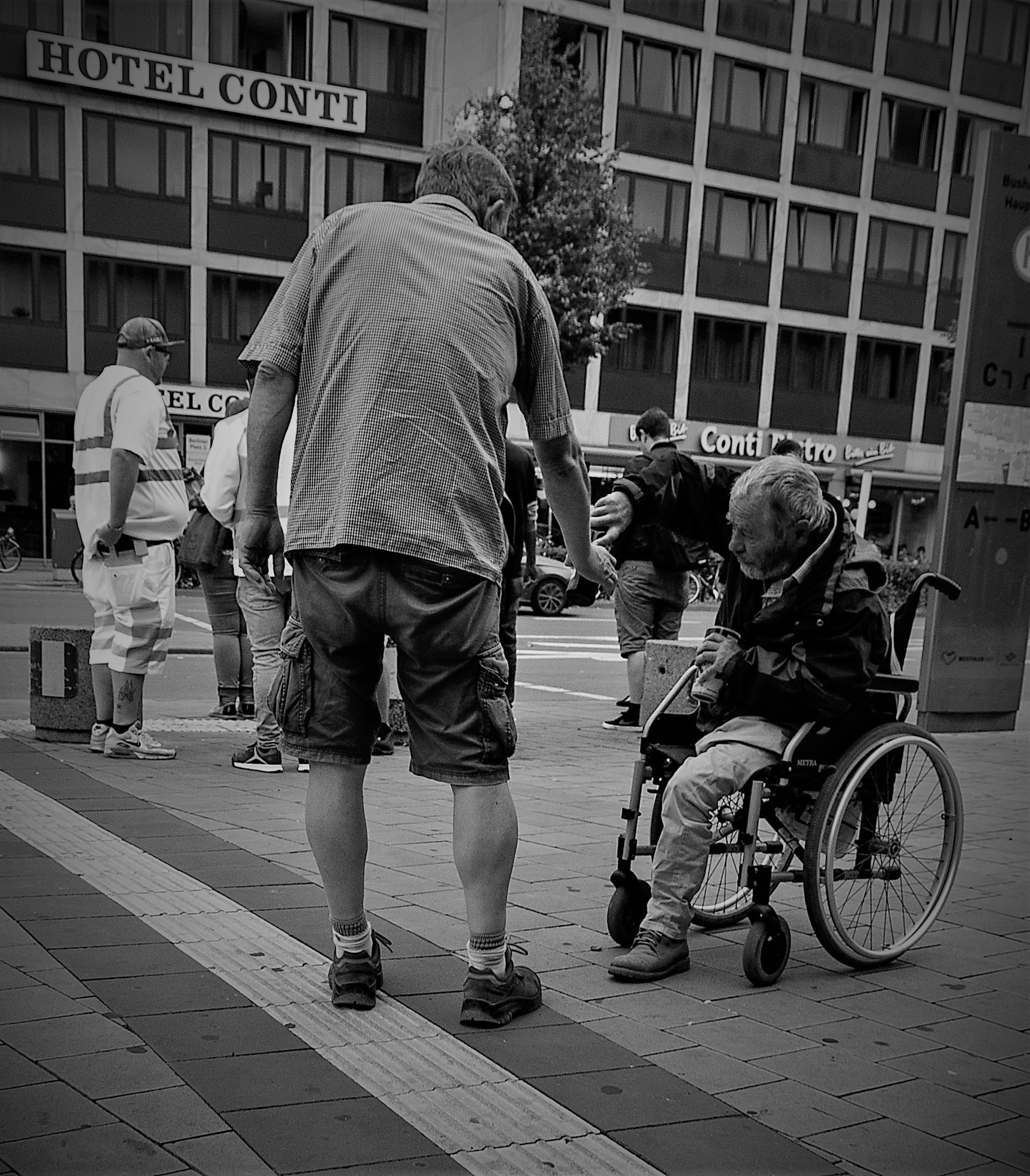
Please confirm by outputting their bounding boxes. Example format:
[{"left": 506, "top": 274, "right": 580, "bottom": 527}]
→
[{"left": 520, "top": 554, "right": 599, "bottom": 617}]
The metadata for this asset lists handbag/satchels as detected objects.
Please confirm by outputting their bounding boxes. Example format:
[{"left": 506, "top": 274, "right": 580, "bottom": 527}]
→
[
  {"left": 654, "top": 528, "right": 710, "bottom": 574},
  {"left": 177, "top": 507, "right": 227, "bottom": 570}
]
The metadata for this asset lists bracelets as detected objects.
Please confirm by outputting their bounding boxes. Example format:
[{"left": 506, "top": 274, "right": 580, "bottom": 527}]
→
[{"left": 106, "top": 522, "right": 125, "bottom": 533}]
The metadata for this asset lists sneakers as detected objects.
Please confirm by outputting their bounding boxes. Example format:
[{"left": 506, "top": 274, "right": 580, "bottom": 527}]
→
[
  {"left": 328, "top": 928, "right": 384, "bottom": 1010},
  {"left": 90, "top": 720, "right": 176, "bottom": 760},
  {"left": 460, "top": 944, "right": 541, "bottom": 1026}
]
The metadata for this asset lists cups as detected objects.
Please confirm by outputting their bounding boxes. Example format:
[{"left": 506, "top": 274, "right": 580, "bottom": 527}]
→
[{"left": 690, "top": 625, "right": 741, "bottom": 704}]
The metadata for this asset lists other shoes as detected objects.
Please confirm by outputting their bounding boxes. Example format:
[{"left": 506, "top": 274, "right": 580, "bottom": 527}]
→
[
  {"left": 603, "top": 700, "right": 643, "bottom": 732},
  {"left": 208, "top": 701, "right": 238, "bottom": 720},
  {"left": 237, "top": 700, "right": 255, "bottom": 718},
  {"left": 231, "top": 741, "right": 283, "bottom": 773},
  {"left": 607, "top": 926, "right": 691, "bottom": 980},
  {"left": 297, "top": 758, "right": 311, "bottom": 771}
]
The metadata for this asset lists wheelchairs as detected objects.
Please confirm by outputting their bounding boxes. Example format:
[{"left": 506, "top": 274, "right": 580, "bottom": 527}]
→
[{"left": 606, "top": 571, "right": 965, "bottom": 988}]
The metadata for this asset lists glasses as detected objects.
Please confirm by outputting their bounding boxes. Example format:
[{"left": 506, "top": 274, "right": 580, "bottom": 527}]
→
[{"left": 152, "top": 345, "right": 172, "bottom": 362}]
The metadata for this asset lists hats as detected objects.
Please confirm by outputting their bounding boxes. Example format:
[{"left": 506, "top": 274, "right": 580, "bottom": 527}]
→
[{"left": 116, "top": 315, "right": 185, "bottom": 349}]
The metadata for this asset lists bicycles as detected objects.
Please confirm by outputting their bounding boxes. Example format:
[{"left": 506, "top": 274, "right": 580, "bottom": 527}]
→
[
  {"left": 71, "top": 546, "right": 182, "bottom": 585},
  {"left": 0, "top": 527, "right": 22, "bottom": 573},
  {"left": 688, "top": 560, "right": 725, "bottom": 604}
]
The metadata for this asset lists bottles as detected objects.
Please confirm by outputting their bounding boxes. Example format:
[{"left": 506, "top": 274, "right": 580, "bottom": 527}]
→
[{"left": 565, "top": 525, "right": 612, "bottom": 607}]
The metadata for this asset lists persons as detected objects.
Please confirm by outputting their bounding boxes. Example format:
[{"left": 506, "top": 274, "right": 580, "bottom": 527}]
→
[
  {"left": 182, "top": 136, "right": 620, "bottom": 1028},
  {"left": 71, "top": 316, "right": 190, "bottom": 760},
  {"left": 603, "top": 407, "right": 692, "bottom": 730},
  {"left": 898, "top": 543, "right": 932, "bottom": 564},
  {"left": 591, "top": 454, "right": 895, "bottom": 981},
  {"left": 771, "top": 440, "right": 806, "bottom": 463}
]
